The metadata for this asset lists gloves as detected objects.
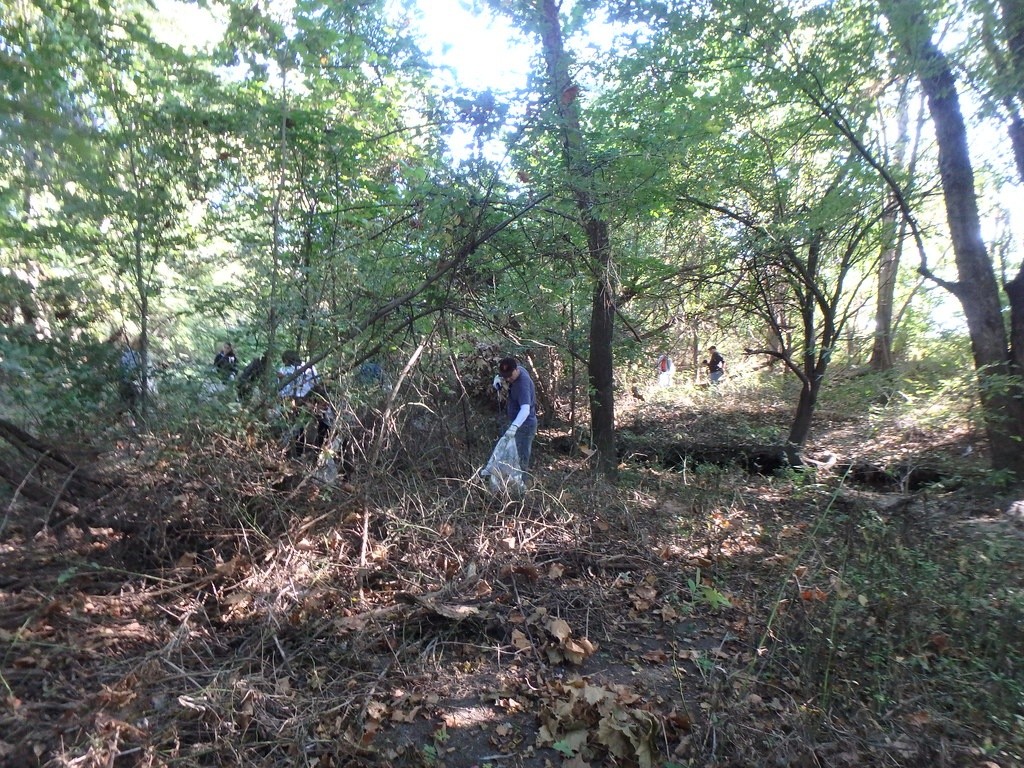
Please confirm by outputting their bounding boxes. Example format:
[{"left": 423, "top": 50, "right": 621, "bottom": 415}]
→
[
  {"left": 505, "top": 425, "right": 517, "bottom": 440},
  {"left": 493, "top": 375, "right": 504, "bottom": 391}
]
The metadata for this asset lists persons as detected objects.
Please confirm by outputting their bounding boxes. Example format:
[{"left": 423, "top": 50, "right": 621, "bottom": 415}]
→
[
  {"left": 277, "top": 350, "right": 320, "bottom": 417},
  {"left": 704, "top": 346, "right": 726, "bottom": 384},
  {"left": 659, "top": 352, "right": 673, "bottom": 387},
  {"left": 214, "top": 341, "right": 239, "bottom": 391},
  {"left": 118, "top": 333, "right": 150, "bottom": 401},
  {"left": 236, "top": 349, "right": 272, "bottom": 408},
  {"left": 493, "top": 357, "right": 537, "bottom": 484},
  {"left": 356, "top": 358, "right": 386, "bottom": 393}
]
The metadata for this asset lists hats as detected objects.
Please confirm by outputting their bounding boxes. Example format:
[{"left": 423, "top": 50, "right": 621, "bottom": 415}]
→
[
  {"left": 499, "top": 358, "right": 516, "bottom": 378},
  {"left": 708, "top": 346, "right": 716, "bottom": 350}
]
